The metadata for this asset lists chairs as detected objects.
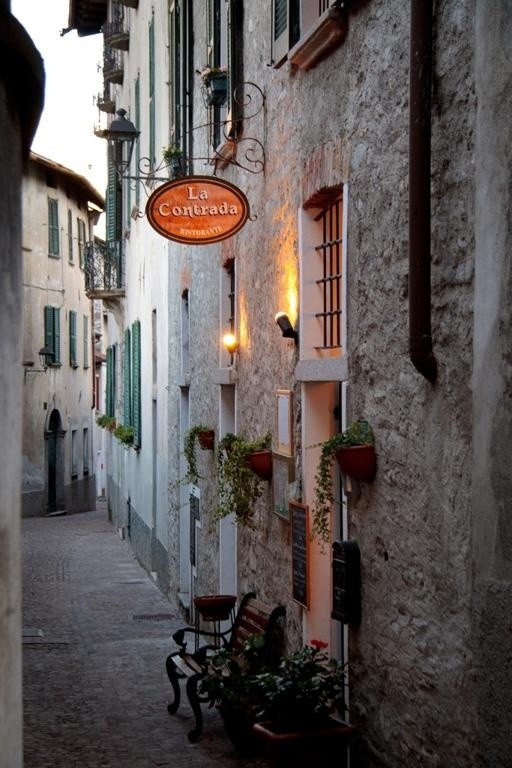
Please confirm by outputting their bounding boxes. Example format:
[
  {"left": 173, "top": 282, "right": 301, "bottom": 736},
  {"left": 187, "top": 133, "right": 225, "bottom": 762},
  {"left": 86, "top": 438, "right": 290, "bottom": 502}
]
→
[{"left": 165, "top": 593, "right": 286, "bottom": 743}]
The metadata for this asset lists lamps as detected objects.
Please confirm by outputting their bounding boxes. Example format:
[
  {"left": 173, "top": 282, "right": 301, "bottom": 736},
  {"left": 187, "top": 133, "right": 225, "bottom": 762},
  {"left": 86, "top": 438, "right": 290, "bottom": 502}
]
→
[
  {"left": 103, "top": 107, "right": 165, "bottom": 183},
  {"left": 274, "top": 312, "right": 296, "bottom": 339},
  {"left": 22, "top": 342, "right": 56, "bottom": 376},
  {"left": 222, "top": 334, "right": 240, "bottom": 353}
]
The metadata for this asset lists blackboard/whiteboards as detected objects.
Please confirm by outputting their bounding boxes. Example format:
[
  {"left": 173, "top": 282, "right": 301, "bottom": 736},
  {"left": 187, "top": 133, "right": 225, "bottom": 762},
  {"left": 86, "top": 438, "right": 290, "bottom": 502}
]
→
[{"left": 289, "top": 500, "right": 311, "bottom": 611}]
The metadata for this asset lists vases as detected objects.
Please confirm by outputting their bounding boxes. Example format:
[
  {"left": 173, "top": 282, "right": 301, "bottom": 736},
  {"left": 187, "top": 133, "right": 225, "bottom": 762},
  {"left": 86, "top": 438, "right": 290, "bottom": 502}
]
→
[
  {"left": 250, "top": 715, "right": 358, "bottom": 766},
  {"left": 197, "top": 593, "right": 237, "bottom": 622}
]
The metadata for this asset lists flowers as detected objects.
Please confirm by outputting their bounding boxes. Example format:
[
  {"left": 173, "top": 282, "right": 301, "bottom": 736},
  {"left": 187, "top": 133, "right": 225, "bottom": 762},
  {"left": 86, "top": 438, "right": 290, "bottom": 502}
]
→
[{"left": 259, "top": 639, "right": 347, "bottom": 730}]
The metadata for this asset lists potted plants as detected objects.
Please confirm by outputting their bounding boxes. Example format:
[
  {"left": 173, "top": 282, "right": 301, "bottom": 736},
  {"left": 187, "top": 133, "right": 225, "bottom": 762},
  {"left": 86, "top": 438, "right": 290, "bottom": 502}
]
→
[
  {"left": 184, "top": 425, "right": 275, "bottom": 525},
  {"left": 311, "top": 420, "right": 377, "bottom": 541},
  {"left": 95, "top": 414, "right": 134, "bottom": 444},
  {"left": 163, "top": 144, "right": 186, "bottom": 180},
  {"left": 207, "top": 637, "right": 279, "bottom": 743},
  {"left": 198, "top": 65, "right": 227, "bottom": 107}
]
